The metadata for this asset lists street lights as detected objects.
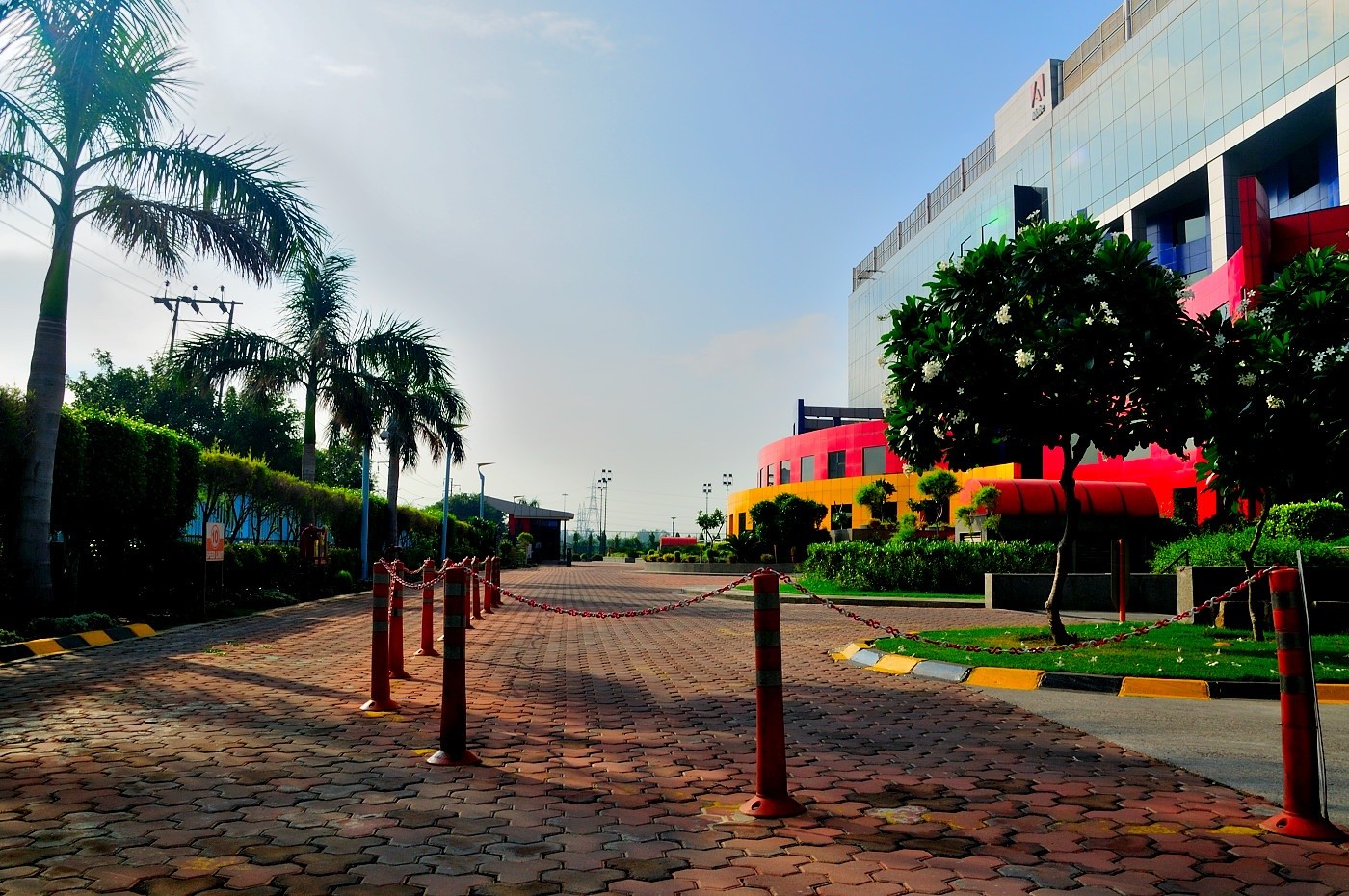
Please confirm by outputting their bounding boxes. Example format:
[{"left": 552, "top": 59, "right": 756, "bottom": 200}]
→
[
  {"left": 597, "top": 479, "right": 606, "bottom": 546},
  {"left": 441, "top": 422, "right": 469, "bottom": 564},
  {"left": 671, "top": 517, "right": 676, "bottom": 537},
  {"left": 722, "top": 474, "right": 733, "bottom": 514},
  {"left": 477, "top": 463, "right": 493, "bottom": 520},
  {"left": 703, "top": 483, "right": 712, "bottom": 543},
  {"left": 562, "top": 494, "right": 568, "bottom": 561},
  {"left": 601, "top": 469, "right": 612, "bottom": 556}
]
